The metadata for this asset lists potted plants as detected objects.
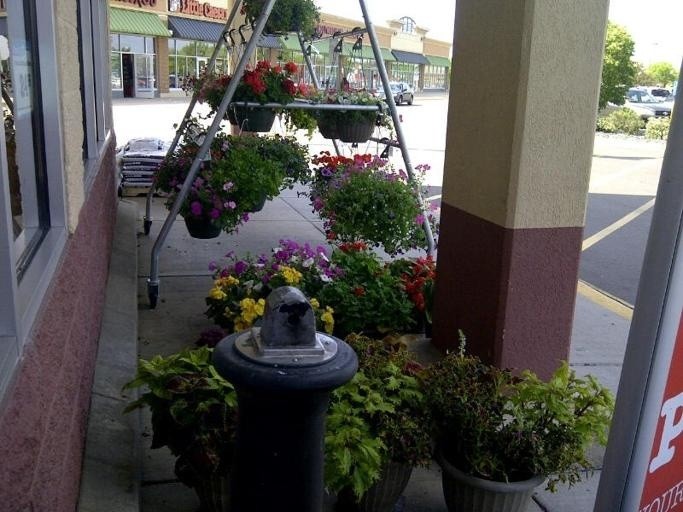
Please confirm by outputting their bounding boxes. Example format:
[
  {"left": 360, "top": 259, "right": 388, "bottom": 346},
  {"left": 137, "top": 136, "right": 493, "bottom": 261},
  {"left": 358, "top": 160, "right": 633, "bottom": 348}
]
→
[
  {"left": 431, "top": 329, "right": 617, "bottom": 512},
  {"left": 240, "top": 0, "right": 320, "bottom": 40},
  {"left": 323, "top": 332, "right": 430, "bottom": 512},
  {"left": 120, "top": 342, "right": 239, "bottom": 512}
]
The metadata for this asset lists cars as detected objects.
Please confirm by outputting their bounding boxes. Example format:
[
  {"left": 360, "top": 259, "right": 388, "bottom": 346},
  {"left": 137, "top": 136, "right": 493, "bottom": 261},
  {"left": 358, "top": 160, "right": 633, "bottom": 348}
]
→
[
  {"left": 606, "top": 86, "right": 674, "bottom": 129},
  {"left": 378, "top": 81, "right": 413, "bottom": 105}
]
